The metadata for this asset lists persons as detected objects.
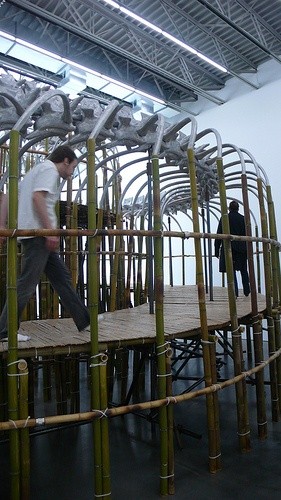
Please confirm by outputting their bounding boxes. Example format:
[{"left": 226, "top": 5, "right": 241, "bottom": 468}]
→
[
  {"left": 216, "top": 201, "right": 253, "bottom": 300},
  {"left": 0, "top": 145, "right": 105, "bottom": 343}
]
